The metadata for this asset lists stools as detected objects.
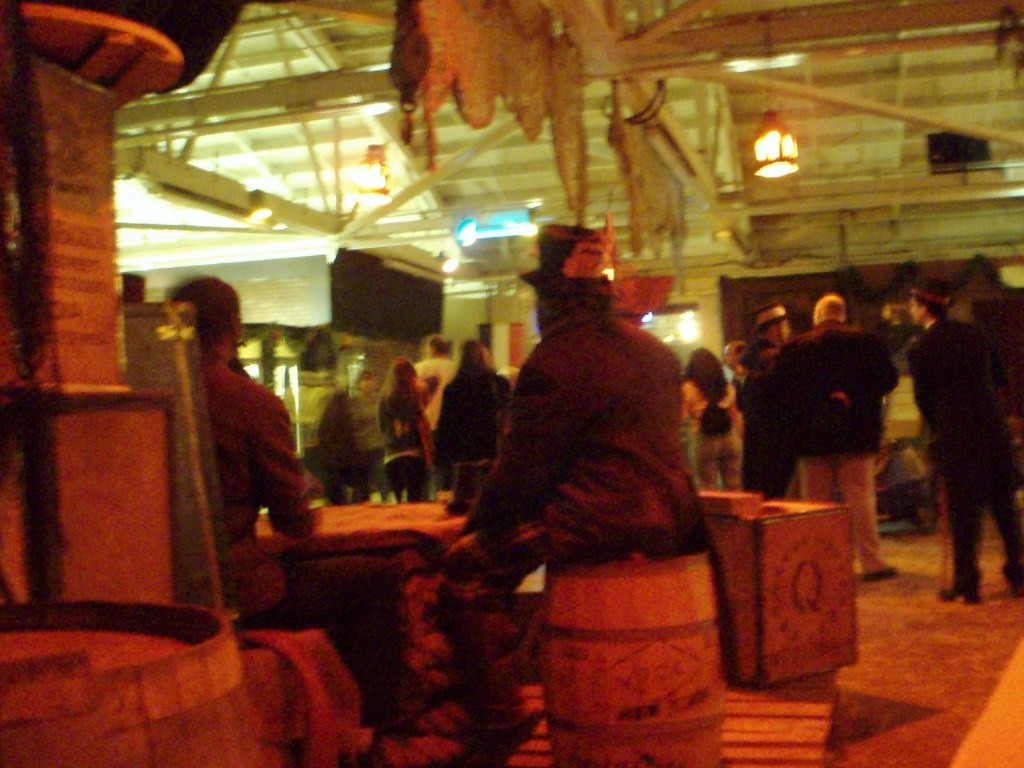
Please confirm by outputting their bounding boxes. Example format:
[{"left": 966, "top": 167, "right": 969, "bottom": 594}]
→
[{"left": 538, "top": 551, "right": 725, "bottom": 768}]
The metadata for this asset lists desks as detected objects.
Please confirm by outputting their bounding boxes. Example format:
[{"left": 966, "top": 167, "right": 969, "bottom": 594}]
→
[{"left": 227, "top": 501, "right": 468, "bottom": 722}]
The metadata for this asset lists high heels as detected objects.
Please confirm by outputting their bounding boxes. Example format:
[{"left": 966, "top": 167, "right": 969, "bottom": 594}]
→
[
  {"left": 940, "top": 588, "right": 979, "bottom": 602},
  {"left": 1003, "top": 564, "right": 1024, "bottom": 592}
]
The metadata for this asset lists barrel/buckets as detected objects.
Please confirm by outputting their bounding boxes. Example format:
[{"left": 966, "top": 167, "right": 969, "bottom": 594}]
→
[
  {"left": 542, "top": 541, "right": 725, "bottom": 768},
  {"left": 0, "top": 602, "right": 265, "bottom": 768},
  {"left": 291, "top": 554, "right": 410, "bottom": 722}
]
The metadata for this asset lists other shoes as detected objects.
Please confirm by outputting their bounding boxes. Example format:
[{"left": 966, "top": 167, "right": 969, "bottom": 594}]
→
[{"left": 865, "top": 569, "right": 896, "bottom": 581}]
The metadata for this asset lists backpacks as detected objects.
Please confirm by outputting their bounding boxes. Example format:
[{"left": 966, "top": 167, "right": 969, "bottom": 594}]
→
[{"left": 701, "top": 400, "right": 731, "bottom": 435}]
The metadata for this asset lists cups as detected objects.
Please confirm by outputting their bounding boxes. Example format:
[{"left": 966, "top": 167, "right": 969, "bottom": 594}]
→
[{"left": 451, "top": 462, "right": 480, "bottom": 507}]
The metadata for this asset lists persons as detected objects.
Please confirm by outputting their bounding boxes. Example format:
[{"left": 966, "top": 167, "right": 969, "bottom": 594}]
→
[
  {"left": 433, "top": 339, "right": 511, "bottom": 504},
  {"left": 908, "top": 281, "right": 1024, "bottom": 603},
  {"left": 175, "top": 276, "right": 411, "bottom": 736},
  {"left": 724, "top": 339, "right": 751, "bottom": 411},
  {"left": 681, "top": 347, "right": 743, "bottom": 494},
  {"left": 784, "top": 294, "right": 900, "bottom": 582},
  {"left": 415, "top": 335, "right": 456, "bottom": 432},
  {"left": 376, "top": 360, "right": 434, "bottom": 503},
  {"left": 345, "top": 369, "right": 384, "bottom": 504},
  {"left": 746, "top": 299, "right": 793, "bottom": 501},
  {"left": 445, "top": 219, "right": 705, "bottom": 768}
]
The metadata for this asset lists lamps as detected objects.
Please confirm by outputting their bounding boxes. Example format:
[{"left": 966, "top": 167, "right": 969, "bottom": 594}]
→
[{"left": 751, "top": 32, "right": 800, "bottom": 179}]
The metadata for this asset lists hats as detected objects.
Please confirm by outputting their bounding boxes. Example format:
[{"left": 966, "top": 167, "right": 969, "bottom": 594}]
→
[
  {"left": 520, "top": 225, "right": 618, "bottom": 297},
  {"left": 751, "top": 301, "right": 796, "bottom": 335},
  {"left": 909, "top": 277, "right": 955, "bottom": 316}
]
